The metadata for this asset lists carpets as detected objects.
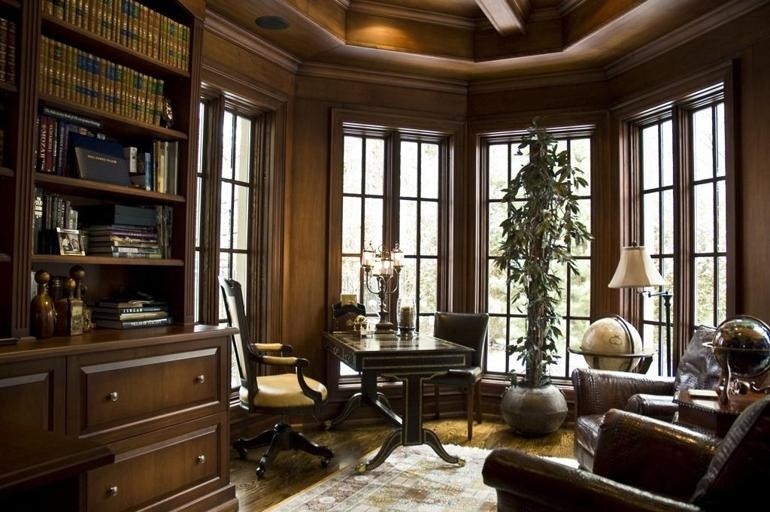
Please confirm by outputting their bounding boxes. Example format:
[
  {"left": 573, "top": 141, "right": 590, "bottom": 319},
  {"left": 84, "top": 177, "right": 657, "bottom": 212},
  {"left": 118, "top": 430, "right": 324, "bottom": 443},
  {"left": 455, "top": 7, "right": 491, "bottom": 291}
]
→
[{"left": 259, "top": 441, "right": 578, "bottom": 511}]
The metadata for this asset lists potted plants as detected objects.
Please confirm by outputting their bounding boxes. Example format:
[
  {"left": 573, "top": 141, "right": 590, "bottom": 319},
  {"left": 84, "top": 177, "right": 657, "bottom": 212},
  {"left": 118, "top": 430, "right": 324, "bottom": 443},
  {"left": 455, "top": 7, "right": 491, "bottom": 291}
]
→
[{"left": 490, "top": 112, "right": 597, "bottom": 443}]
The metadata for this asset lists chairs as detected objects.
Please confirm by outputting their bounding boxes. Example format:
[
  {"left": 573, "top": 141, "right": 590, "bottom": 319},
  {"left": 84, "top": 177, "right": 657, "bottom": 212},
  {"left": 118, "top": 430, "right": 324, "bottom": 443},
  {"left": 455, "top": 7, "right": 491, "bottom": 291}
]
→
[
  {"left": 429, "top": 312, "right": 489, "bottom": 450},
  {"left": 217, "top": 277, "right": 339, "bottom": 479}
]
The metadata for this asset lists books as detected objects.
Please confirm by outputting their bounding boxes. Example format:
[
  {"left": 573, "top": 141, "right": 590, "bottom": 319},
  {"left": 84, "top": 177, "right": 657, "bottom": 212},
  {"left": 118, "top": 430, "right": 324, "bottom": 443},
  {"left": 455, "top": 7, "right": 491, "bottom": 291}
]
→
[
  {"left": 34, "top": 189, "right": 174, "bottom": 260},
  {"left": 36, "top": 108, "right": 179, "bottom": 197},
  {"left": 91, "top": 302, "right": 171, "bottom": 329},
  {"left": 2, "top": 1, "right": 21, "bottom": 88},
  {"left": 41, "top": 1, "right": 191, "bottom": 126}
]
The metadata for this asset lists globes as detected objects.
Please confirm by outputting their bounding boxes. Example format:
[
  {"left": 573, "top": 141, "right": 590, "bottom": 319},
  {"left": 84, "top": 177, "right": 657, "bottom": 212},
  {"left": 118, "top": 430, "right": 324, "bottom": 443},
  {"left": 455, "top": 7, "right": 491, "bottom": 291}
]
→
[
  {"left": 566, "top": 314, "right": 654, "bottom": 375},
  {"left": 700, "top": 315, "right": 770, "bottom": 406}
]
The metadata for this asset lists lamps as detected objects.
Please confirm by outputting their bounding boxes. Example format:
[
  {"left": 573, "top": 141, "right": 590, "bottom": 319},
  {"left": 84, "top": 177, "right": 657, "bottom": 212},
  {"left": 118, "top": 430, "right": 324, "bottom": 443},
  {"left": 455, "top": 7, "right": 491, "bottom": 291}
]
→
[
  {"left": 362, "top": 240, "right": 406, "bottom": 336},
  {"left": 608, "top": 242, "right": 674, "bottom": 377}
]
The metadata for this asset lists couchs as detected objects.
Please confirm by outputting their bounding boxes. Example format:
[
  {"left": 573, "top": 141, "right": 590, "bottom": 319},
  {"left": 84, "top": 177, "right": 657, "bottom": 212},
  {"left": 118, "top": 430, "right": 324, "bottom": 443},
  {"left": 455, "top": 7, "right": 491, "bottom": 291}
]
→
[
  {"left": 480, "top": 399, "right": 767, "bottom": 510},
  {"left": 571, "top": 320, "right": 754, "bottom": 472}
]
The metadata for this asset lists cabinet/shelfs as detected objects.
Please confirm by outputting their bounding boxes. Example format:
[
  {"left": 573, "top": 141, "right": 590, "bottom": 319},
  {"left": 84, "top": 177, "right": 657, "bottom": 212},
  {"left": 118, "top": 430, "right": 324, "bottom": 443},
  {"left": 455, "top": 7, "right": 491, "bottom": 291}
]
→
[
  {"left": 65, "top": 331, "right": 239, "bottom": 511},
  {"left": 0, "top": 355, "right": 65, "bottom": 438},
  {"left": 0, "top": 0, "right": 197, "bottom": 353}
]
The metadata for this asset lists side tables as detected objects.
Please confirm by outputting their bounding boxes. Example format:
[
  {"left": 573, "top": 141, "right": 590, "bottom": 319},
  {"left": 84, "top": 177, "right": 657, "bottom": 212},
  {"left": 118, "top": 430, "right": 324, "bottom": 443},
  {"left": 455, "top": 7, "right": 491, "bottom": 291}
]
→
[{"left": 673, "top": 388, "right": 770, "bottom": 442}]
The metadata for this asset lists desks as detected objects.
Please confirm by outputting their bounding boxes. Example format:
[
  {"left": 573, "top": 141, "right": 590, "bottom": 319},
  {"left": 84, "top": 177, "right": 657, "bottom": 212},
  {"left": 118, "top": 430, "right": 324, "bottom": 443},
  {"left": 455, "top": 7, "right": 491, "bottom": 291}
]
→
[
  {"left": 323, "top": 323, "right": 470, "bottom": 473},
  {"left": 0, "top": 426, "right": 115, "bottom": 511}
]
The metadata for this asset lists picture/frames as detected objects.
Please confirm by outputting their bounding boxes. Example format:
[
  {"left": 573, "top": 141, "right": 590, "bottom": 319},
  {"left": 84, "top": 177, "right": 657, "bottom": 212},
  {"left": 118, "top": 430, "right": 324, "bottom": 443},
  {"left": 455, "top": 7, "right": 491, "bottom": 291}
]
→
[{"left": 55, "top": 226, "right": 85, "bottom": 257}]
